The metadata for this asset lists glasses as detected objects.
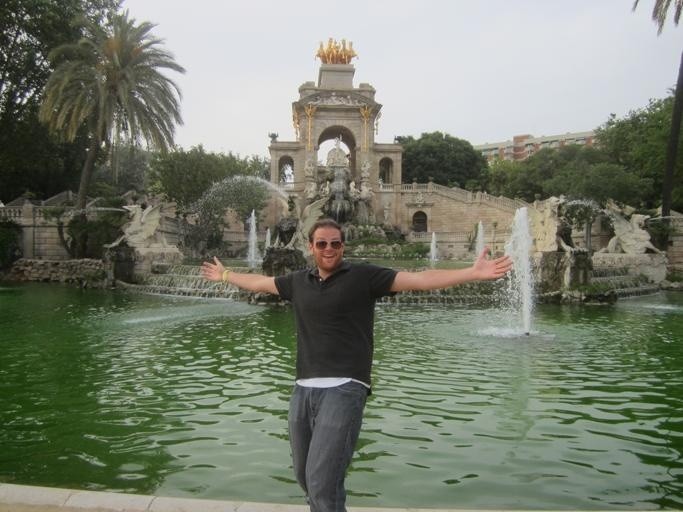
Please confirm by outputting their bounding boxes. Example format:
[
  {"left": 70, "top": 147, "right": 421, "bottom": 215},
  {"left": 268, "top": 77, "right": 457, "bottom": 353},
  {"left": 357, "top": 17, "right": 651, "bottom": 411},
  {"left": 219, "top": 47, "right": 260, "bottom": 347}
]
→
[{"left": 315, "top": 240, "right": 342, "bottom": 249}]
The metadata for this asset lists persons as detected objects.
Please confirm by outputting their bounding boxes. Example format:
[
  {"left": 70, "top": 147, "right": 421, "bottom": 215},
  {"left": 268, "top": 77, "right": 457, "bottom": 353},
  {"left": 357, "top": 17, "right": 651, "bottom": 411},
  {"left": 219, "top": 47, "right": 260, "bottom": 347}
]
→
[{"left": 201, "top": 220, "right": 512, "bottom": 512}]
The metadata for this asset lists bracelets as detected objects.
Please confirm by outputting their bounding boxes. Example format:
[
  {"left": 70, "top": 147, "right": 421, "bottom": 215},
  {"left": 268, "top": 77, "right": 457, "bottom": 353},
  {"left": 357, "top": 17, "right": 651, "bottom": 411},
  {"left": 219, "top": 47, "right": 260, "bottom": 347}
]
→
[{"left": 222, "top": 269, "right": 230, "bottom": 283}]
